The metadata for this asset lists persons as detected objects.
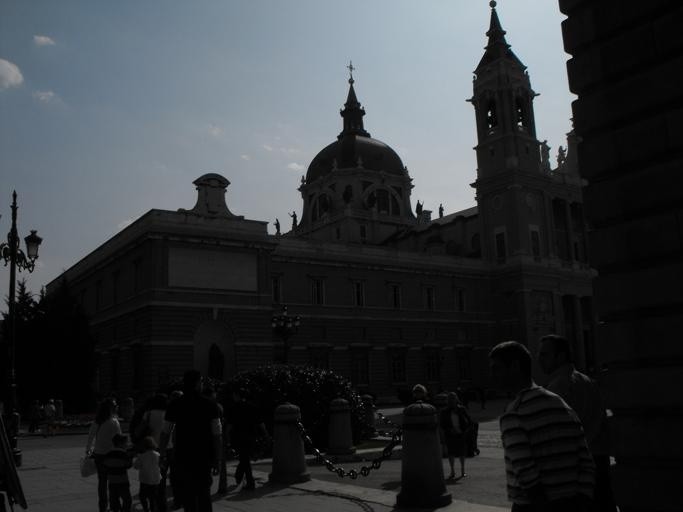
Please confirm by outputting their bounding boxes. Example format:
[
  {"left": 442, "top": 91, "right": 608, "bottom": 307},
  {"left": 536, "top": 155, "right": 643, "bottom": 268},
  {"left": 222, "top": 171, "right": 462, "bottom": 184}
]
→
[
  {"left": 28, "top": 401, "right": 44, "bottom": 432},
  {"left": 488, "top": 340, "right": 599, "bottom": 511},
  {"left": 536, "top": 333, "right": 617, "bottom": 512},
  {"left": 44, "top": 398, "right": 57, "bottom": 425},
  {"left": 439, "top": 391, "right": 474, "bottom": 480},
  {"left": 84, "top": 370, "right": 268, "bottom": 511}
]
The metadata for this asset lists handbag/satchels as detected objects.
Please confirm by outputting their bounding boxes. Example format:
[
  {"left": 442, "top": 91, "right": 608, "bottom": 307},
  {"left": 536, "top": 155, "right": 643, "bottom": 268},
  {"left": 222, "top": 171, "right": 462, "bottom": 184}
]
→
[{"left": 79, "top": 453, "right": 98, "bottom": 477}]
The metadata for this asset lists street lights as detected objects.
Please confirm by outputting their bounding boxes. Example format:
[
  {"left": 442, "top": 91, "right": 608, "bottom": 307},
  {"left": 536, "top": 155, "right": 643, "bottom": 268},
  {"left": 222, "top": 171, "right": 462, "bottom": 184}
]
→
[
  {"left": 269, "top": 304, "right": 304, "bottom": 366},
  {"left": 0, "top": 187, "right": 43, "bottom": 469}
]
